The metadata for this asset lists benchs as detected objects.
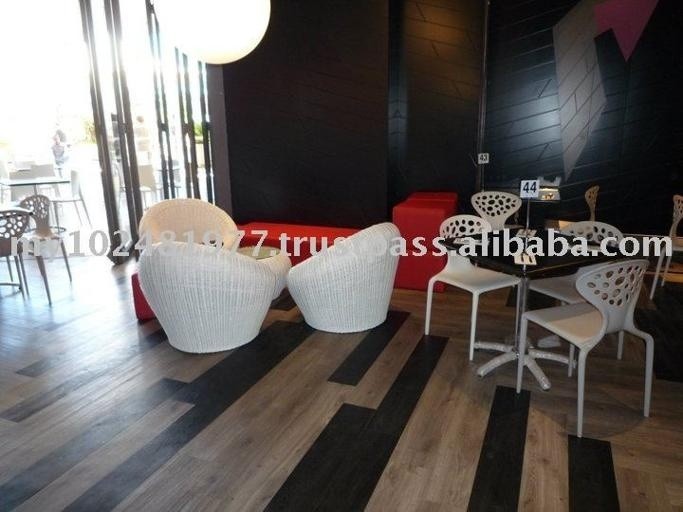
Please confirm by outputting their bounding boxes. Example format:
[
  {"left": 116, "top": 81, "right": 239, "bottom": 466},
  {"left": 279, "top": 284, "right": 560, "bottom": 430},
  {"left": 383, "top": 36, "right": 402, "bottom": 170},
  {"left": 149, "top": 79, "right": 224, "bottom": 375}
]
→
[
  {"left": 240, "top": 216, "right": 367, "bottom": 319},
  {"left": 127, "top": 219, "right": 244, "bottom": 322}
]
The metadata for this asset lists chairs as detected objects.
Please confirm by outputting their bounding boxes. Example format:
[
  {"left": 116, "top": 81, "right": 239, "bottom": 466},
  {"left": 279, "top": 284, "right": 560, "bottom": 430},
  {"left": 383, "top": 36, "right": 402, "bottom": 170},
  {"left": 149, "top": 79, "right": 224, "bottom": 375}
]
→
[
  {"left": 583, "top": 184, "right": 599, "bottom": 223},
  {"left": 135, "top": 197, "right": 238, "bottom": 247},
  {"left": 132, "top": 239, "right": 275, "bottom": 355},
  {"left": 468, "top": 190, "right": 525, "bottom": 234},
  {"left": 650, "top": 192, "right": 682, "bottom": 300},
  {"left": 285, "top": 219, "right": 405, "bottom": 334},
  {"left": 0, "top": 153, "right": 180, "bottom": 308},
  {"left": 421, "top": 212, "right": 523, "bottom": 362},
  {"left": 518, "top": 219, "right": 630, "bottom": 379},
  {"left": 511, "top": 255, "right": 657, "bottom": 441}
]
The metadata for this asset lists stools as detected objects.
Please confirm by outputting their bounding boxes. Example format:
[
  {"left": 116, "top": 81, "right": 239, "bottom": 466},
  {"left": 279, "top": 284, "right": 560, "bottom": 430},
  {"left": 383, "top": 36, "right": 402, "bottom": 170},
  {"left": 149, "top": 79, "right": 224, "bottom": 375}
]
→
[
  {"left": 411, "top": 189, "right": 457, "bottom": 213},
  {"left": 389, "top": 199, "right": 449, "bottom": 296}
]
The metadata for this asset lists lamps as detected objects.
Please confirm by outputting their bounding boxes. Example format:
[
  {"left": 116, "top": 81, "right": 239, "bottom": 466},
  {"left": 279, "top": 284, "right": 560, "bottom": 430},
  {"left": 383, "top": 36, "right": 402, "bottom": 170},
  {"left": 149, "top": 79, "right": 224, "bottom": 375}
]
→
[{"left": 151, "top": 0, "right": 276, "bottom": 68}]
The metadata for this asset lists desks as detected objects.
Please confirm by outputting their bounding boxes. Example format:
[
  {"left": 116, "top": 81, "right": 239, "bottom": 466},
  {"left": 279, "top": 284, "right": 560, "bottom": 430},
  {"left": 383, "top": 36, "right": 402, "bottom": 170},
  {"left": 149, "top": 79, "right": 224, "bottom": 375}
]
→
[
  {"left": 237, "top": 244, "right": 290, "bottom": 308},
  {"left": 433, "top": 221, "right": 632, "bottom": 395}
]
